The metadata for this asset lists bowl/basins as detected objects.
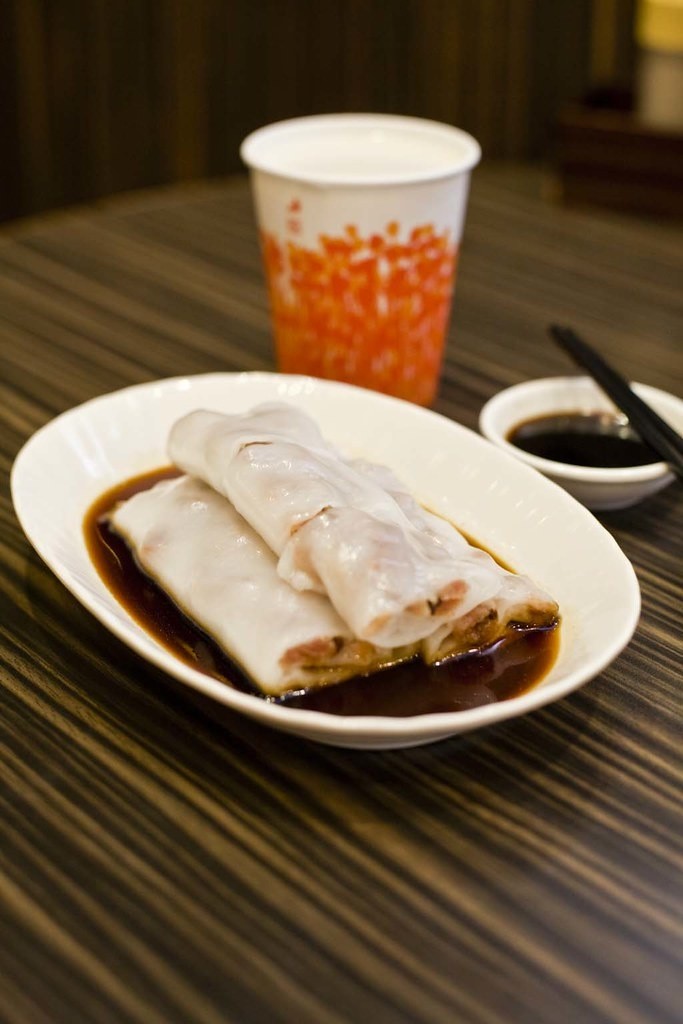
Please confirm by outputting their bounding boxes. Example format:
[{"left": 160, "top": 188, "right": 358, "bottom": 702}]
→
[{"left": 478, "top": 376, "right": 683, "bottom": 510}]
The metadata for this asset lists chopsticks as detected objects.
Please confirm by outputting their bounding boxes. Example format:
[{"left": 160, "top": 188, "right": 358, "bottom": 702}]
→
[{"left": 548, "top": 323, "right": 683, "bottom": 479}]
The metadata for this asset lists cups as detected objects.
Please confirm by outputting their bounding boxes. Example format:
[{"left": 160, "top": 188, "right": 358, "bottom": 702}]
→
[{"left": 241, "top": 112, "right": 482, "bottom": 408}]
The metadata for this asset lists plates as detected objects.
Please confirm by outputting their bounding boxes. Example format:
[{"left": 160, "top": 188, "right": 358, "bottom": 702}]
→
[{"left": 9, "top": 371, "right": 641, "bottom": 750}]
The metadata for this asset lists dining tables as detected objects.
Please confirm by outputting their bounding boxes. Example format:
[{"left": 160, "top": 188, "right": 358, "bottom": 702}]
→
[{"left": 0, "top": 181, "right": 682, "bottom": 1024}]
